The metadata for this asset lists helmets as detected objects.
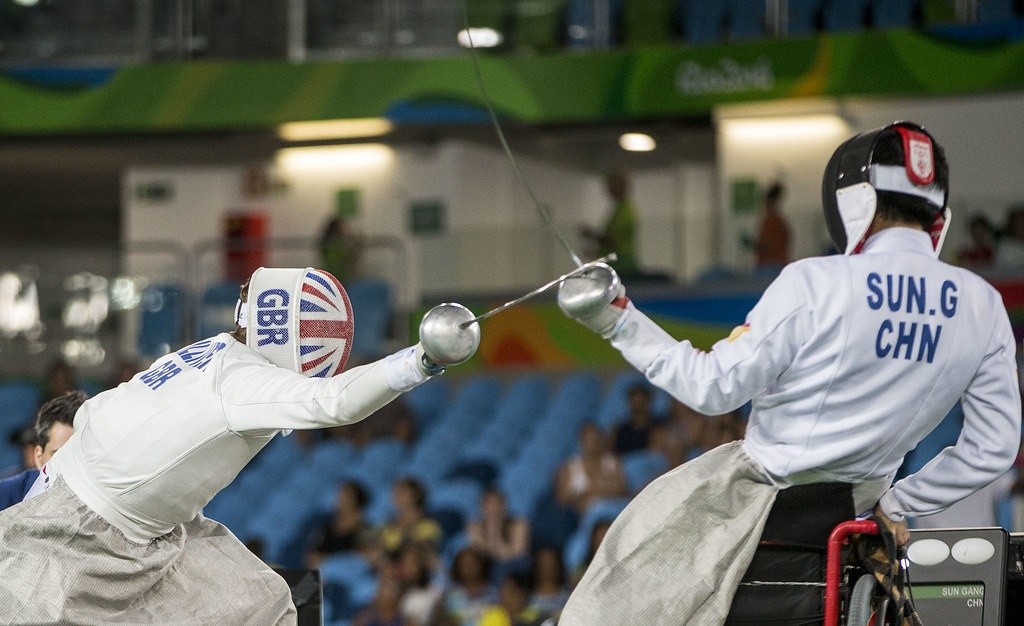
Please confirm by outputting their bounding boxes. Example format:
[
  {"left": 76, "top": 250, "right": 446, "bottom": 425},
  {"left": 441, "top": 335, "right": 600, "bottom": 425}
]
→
[
  {"left": 246, "top": 265, "right": 355, "bottom": 378},
  {"left": 821, "top": 121, "right": 948, "bottom": 248}
]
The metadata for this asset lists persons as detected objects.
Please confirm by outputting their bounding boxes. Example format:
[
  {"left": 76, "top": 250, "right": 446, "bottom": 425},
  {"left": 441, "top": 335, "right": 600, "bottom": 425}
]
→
[{"left": 0, "top": 121, "right": 1024, "bottom": 626}]
